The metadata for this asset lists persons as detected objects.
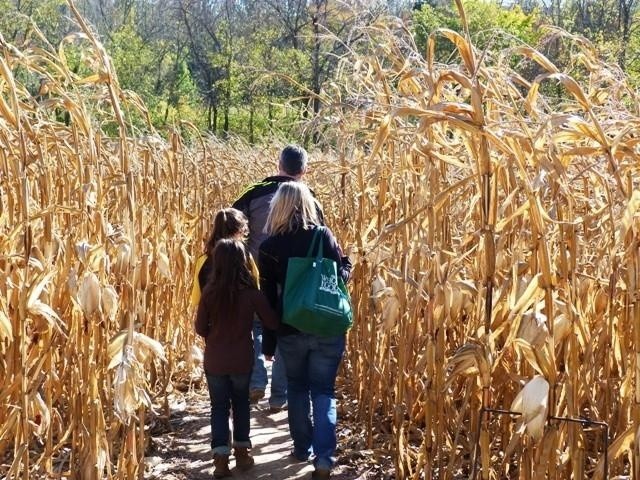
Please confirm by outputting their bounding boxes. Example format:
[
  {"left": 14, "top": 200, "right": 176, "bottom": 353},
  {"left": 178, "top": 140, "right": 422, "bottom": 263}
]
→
[
  {"left": 258, "top": 180, "right": 353, "bottom": 479},
  {"left": 231, "top": 143, "right": 325, "bottom": 413},
  {"left": 194, "top": 207, "right": 259, "bottom": 307},
  {"left": 194, "top": 238, "right": 262, "bottom": 479}
]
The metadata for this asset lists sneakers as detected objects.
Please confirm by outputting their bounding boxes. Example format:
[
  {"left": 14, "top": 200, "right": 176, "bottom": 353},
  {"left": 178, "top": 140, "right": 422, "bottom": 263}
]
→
[
  {"left": 249, "top": 388, "right": 265, "bottom": 401},
  {"left": 270, "top": 404, "right": 288, "bottom": 412},
  {"left": 312, "top": 471, "right": 330, "bottom": 480},
  {"left": 291, "top": 450, "right": 308, "bottom": 461},
  {"left": 265, "top": 355, "right": 273, "bottom": 361},
  {"left": 213, "top": 447, "right": 254, "bottom": 478}
]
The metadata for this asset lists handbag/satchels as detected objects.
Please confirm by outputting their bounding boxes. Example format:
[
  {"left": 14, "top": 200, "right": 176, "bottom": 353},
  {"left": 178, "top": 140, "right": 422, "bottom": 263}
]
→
[{"left": 282, "top": 226, "right": 353, "bottom": 337}]
